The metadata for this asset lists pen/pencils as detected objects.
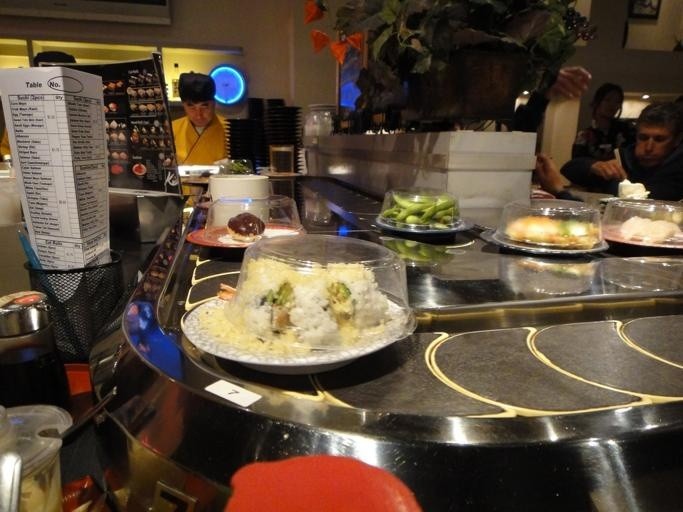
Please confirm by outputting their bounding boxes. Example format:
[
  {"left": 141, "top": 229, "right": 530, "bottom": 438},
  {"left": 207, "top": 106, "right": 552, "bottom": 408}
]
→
[{"left": 16, "top": 227, "right": 88, "bottom": 360}]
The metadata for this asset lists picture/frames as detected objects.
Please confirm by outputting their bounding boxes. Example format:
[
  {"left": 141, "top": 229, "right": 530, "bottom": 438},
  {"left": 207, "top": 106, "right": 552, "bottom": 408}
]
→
[{"left": 627, "top": 0, "right": 662, "bottom": 19}]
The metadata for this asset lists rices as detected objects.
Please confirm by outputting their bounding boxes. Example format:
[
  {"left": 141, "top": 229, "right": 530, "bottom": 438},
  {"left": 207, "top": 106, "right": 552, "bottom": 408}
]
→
[{"left": 200, "top": 258, "right": 391, "bottom": 357}]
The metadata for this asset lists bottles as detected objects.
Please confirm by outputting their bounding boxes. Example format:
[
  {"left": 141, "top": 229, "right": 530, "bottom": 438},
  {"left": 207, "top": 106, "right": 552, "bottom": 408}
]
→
[{"left": 0, "top": 291, "right": 71, "bottom": 406}]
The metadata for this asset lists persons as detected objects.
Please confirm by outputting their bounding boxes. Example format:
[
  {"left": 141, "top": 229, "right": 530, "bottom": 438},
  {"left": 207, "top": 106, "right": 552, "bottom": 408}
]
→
[
  {"left": 515, "top": 65, "right": 682, "bottom": 204},
  {"left": 1, "top": 50, "right": 77, "bottom": 162},
  {"left": 172, "top": 71, "right": 230, "bottom": 206}
]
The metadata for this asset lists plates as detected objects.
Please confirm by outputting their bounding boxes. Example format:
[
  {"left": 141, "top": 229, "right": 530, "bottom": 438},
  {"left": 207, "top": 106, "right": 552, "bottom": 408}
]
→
[{"left": 225, "top": 107, "right": 303, "bottom": 174}]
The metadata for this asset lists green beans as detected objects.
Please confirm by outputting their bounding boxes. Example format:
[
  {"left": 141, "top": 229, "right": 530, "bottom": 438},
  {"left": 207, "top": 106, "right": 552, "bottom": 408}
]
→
[{"left": 383, "top": 191, "right": 459, "bottom": 228}]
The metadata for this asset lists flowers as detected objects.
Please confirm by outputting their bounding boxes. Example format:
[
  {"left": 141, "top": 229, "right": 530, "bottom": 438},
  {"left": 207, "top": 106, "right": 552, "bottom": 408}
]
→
[{"left": 302, "top": 0, "right": 617, "bottom": 113}]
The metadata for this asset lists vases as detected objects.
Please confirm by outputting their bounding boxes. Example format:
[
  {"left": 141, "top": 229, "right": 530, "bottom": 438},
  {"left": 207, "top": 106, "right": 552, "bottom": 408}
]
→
[{"left": 401, "top": 49, "right": 526, "bottom": 120}]
